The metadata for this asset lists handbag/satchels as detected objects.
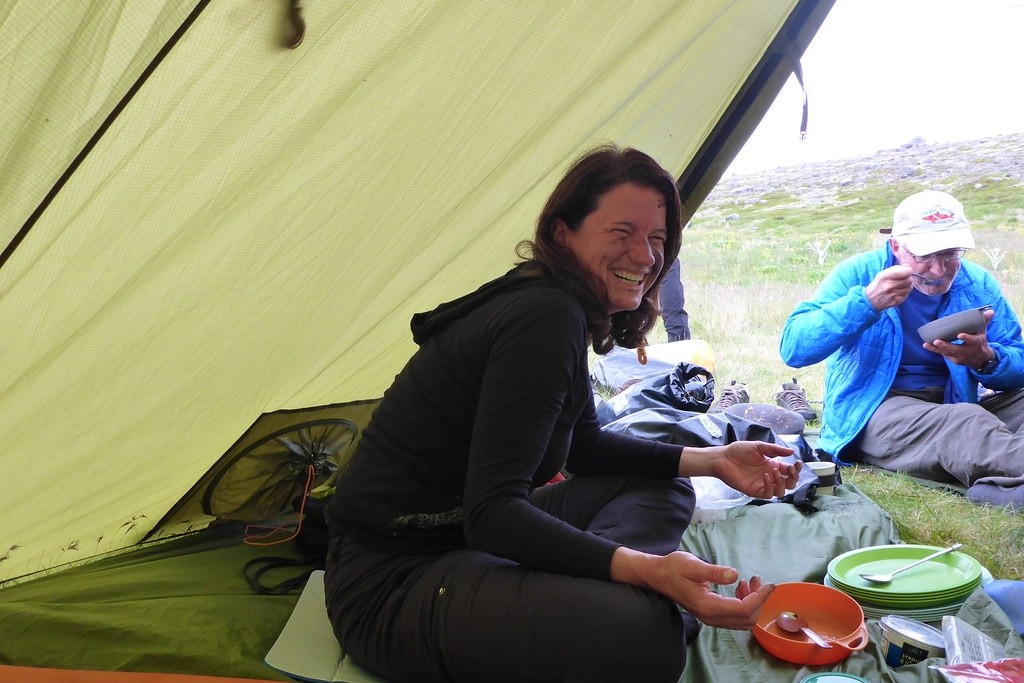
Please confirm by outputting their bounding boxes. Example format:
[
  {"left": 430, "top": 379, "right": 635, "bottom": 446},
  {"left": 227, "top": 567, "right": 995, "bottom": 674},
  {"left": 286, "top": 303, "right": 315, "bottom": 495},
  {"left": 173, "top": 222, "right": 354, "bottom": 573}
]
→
[{"left": 242, "top": 495, "right": 331, "bottom": 594}]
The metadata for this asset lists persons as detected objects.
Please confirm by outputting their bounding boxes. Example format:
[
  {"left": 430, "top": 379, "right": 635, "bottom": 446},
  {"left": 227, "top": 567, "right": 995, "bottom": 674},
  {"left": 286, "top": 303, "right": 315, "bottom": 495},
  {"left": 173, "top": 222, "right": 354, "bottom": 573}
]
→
[
  {"left": 660, "top": 258, "right": 692, "bottom": 342},
  {"left": 323, "top": 145, "right": 803, "bottom": 680},
  {"left": 785, "top": 189, "right": 1024, "bottom": 507}
]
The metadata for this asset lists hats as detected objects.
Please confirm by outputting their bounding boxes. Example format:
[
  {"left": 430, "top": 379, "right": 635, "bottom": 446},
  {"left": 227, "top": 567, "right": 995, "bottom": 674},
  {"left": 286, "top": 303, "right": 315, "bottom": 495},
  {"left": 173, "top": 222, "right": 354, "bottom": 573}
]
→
[{"left": 879, "top": 189, "right": 975, "bottom": 256}]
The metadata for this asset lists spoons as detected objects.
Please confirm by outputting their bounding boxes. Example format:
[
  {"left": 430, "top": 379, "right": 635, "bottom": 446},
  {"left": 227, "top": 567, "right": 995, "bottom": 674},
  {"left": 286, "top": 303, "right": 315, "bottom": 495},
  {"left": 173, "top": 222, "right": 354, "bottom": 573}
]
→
[
  {"left": 860, "top": 543, "right": 962, "bottom": 583},
  {"left": 912, "top": 273, "right": 944, "bottom": 286},
  {"left": 778, "top": 612, "right": 834, "bottom": 649}
]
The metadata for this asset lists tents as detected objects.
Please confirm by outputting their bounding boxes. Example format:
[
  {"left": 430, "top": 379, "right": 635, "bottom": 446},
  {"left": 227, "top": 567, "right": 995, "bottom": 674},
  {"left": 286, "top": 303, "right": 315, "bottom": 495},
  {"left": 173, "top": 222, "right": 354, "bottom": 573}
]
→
[{"left": 0, "top": 0, "right": 835, "bottom": 681}]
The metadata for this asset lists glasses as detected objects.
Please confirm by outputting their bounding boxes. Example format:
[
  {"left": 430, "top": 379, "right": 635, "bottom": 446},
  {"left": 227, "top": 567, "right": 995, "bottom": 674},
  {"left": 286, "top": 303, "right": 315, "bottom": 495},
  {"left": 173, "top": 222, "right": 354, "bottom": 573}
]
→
[{"left": 902, "top": 245, "right": 966, "bottom": 263}]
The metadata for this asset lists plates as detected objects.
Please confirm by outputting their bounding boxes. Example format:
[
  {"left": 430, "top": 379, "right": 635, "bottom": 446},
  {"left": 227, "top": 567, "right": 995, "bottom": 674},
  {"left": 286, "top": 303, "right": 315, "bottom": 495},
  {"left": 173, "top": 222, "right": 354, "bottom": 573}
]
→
[
  {"left": 800, "top": 672, "right": 870, "bottom": 683},
  {"left": 824, "top": 545, "right": 992, "bottom": 623}
]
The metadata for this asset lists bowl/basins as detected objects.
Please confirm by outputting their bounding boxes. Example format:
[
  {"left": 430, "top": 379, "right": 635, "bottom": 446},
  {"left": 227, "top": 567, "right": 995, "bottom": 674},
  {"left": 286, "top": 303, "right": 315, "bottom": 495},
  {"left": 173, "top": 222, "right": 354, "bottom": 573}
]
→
[
  {"left": 753, "top": 582, "right": 868, "bottom": 664},
  {"left": 877, "top": 615, "right": 946, "bottom": 668},
  {"left": 917, "top": 304, "right": 992, "bottom": 344}
]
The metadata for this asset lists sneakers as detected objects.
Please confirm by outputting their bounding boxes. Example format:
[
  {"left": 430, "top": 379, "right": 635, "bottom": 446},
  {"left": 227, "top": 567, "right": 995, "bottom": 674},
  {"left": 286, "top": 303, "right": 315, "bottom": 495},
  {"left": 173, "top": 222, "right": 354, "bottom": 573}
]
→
[
  {"left": 772, "top": 377, "right": 817, "bottom": 422},
  {"left": 708, "top": 380, "right": 750, "bottom": 414}
]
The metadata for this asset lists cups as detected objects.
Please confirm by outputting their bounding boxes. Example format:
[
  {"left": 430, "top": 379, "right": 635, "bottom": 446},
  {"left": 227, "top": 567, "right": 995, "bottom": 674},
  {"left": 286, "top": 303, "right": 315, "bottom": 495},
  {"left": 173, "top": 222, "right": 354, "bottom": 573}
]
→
[{"left": 806, "top": 462, "right": 836, "bottom": 495}]
